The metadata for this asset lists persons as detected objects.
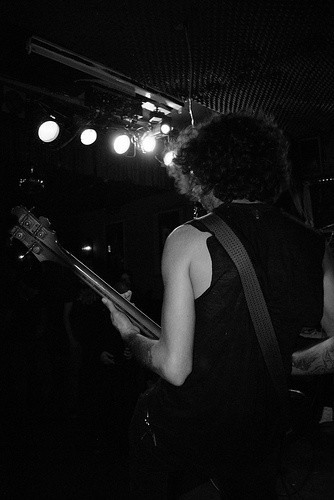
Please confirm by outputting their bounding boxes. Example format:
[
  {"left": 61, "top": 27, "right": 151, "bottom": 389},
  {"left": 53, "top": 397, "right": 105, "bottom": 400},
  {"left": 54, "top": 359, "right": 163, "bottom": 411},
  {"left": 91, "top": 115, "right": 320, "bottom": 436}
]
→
[{"left": 102, "top": 110, "right": 331, "bottom": 500}]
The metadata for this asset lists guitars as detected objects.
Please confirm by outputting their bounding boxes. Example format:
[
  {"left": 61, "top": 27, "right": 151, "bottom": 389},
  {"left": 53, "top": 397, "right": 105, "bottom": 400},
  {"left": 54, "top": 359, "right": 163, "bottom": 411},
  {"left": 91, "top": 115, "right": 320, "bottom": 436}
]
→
[{"left": 5, "top": 202, "right": 317, "bottom": 494}]
